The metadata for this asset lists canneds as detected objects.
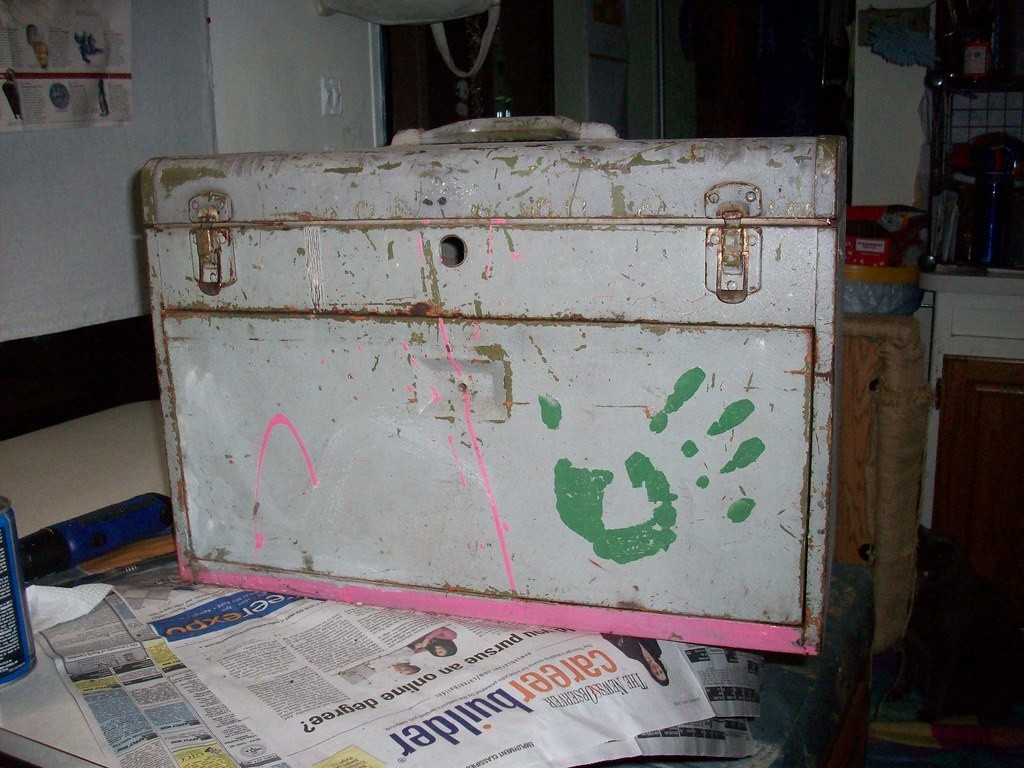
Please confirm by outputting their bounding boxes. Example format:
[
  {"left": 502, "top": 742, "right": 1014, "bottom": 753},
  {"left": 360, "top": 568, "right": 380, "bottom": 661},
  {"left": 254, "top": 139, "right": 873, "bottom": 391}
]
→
[{"left": 0, "top": 496, "right": 38, "bottom": 689}]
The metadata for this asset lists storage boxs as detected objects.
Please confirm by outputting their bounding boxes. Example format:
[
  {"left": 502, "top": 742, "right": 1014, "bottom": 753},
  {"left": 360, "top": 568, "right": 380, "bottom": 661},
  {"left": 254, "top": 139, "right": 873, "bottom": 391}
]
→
[
  {"left": 843, "top": 198, "right": 929, "bottom": 268},
  {"left": 139, "top": 133, "right": 849, "bottom": 657}
]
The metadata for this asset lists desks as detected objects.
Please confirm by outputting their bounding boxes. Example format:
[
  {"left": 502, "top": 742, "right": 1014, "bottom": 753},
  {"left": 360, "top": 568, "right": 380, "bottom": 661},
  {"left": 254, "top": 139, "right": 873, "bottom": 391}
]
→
[{"left": 919, "top": 265, "right": 1024, "bottom": 527}]
